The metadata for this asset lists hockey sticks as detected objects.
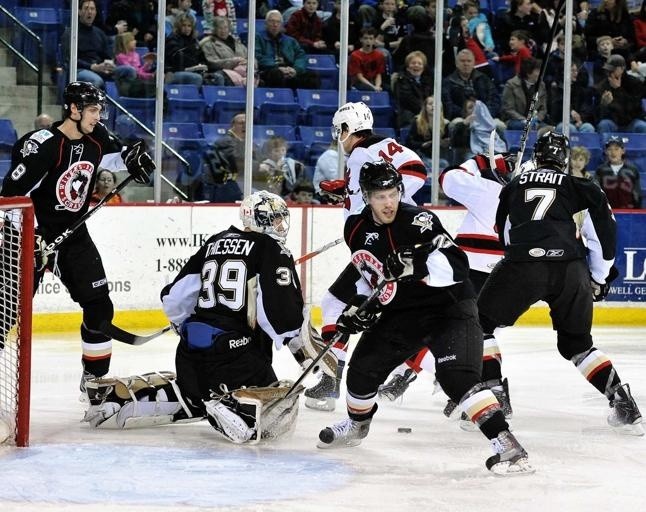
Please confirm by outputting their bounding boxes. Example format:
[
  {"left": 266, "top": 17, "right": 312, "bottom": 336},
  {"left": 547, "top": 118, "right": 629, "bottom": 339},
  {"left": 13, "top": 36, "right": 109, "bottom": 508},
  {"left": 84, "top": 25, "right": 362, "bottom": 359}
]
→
[
  {"left": 286, "top": 278, "right": 386, "bottom": 401},
  {"left": 513, "top": 1, "right": 567, "bottom": 174},
  {"left": 103, "top": 235, "right": 345, "bottom": 345}
]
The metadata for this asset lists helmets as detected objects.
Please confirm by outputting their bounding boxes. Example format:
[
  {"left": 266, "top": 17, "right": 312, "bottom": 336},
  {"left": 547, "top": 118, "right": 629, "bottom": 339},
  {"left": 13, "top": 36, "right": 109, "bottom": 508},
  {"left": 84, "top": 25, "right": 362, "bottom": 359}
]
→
[
  {"left": 332, "top": 102, "right": 373, "bottom": 135},
  {"left": 359, "top": 160, "right": 405, "bottom": 205},
  {"left": 510, "top": 158, "right": 537, "bottom": 183},
  {"left": 531, "top": 130, "right": 571, "bottom": 170},
  {"left": 63, "top": 81, "right": 107, "bottom": 110},
  {"left": 239, "top": 190, "right": 289, "bottom": 243}
]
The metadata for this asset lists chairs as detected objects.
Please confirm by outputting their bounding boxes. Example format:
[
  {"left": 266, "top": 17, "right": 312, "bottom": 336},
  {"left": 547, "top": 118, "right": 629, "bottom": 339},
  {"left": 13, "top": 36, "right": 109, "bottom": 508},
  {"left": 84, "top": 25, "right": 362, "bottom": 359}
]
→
[{"left": 0, "top": 0, "right": 646, "bottom": 213}]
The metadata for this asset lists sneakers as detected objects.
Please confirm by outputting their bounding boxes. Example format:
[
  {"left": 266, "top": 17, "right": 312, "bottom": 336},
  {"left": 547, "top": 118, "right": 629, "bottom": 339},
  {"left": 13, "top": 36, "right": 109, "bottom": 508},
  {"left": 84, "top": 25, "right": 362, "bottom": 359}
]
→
[
  {"left": 607, "top": 397, "right": 642, "bottom": 428},
  {"left": 304, "top": 359, "right": 345, "bottom": 397},
  {"left": 461, "top": 377, "right": 513, "bottom": 422},
  {"left": 205, "top": 400, "right": 255, "bottom": 445},
  {"left": 320, "top": 417, "right": 372, "bottom": 443},
  {"left": 78, "top": 370, "right": 115, "bottom": 393},
  {"left": 485, "top": 430, "right": 528, "bottom": 470},
  {"left": 442, "top": 400, "right": 458, "bottom": 418},
  {"left": 378, "top": 368, "right": 417, "bottom": 401}
]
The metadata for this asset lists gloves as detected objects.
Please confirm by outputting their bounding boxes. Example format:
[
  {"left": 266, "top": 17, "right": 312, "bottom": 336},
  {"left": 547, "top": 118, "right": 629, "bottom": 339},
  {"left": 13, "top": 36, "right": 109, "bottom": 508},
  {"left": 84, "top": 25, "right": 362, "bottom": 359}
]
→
[
  {"left": 32, "top": 226, "right": 55, "bottom": 272},
  {"left": 382, "top": 244, "right": 429, "bottom": 284},
  {"left": 491, "top": 153, "right": 516, "bottom": 174},
  {"left": 121, "top": 138, "right": 156, "bottom": 185},
  {"left": 320, "top": 180, "right": 347, "bottom": 200},
  {"left": 335, "top": 295, "right": 383, "bottom": 335},
  {"left": 589, "top": 278, "right": 611, "bottom": 302}
]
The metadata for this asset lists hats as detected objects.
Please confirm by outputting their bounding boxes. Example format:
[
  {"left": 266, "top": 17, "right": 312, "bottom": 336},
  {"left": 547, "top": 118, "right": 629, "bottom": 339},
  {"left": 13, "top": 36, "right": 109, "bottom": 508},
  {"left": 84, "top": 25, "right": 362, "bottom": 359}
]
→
[
  {"left": 607, "top": 135, "right": 624, "bottom": 148},
  {"left": 601, "top": 54, "right": 625, "bottom": 72}
]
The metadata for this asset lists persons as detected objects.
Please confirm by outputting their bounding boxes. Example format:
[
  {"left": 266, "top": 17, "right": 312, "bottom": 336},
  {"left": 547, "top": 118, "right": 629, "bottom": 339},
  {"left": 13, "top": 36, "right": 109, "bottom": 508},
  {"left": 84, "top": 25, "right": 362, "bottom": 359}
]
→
[
  {"left": 81, "top": 190, "right": 339, "bottom": 443},
  {"left": 34, "top": 0, "right": 646, "bottom": 208},
  {"left": 1, "top": 80, "right": 113, "bottom": 400},
  {"left": 305, "top": 101, "right": 427, "bottom": 402},
  {"left": 460, "top": 130, "right": 644, "bottom": 429},
  {"left": 379, "top": 144, "right": 514, "bottom": 420},
  {"left": 315, "top": 163, "right": 539, "bottom": 470}
]
